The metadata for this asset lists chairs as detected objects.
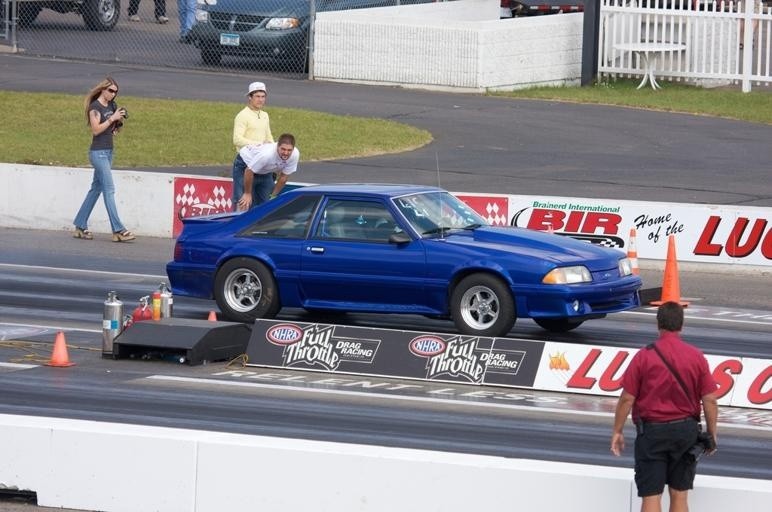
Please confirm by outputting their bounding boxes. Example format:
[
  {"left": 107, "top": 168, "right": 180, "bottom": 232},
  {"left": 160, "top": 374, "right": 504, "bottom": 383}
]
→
[{"left": 328, "top": 207, "right": 366, "bottom": 239}]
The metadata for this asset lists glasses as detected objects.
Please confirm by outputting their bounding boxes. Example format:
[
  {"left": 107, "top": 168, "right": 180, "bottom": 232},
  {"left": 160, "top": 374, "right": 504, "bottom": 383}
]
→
[{"left": 108, "top": 89, "right": 118, "bottom": 94}]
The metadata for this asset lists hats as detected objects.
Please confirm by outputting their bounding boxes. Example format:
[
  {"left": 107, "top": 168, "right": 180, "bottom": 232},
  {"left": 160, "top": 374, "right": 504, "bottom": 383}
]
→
[{"left": 245, "top": 81, "right": 267, "bottom": 95}]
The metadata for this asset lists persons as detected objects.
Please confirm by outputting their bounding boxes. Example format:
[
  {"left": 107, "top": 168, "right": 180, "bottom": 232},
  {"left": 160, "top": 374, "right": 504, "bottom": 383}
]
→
[
  {"left": 735, "top": 1, "right": 764, "bottom": 50},
  {"left": 73, "top": 77, "right": 138, "bottom": 242},
  {"left": 128, "top": 0, "right": 171, "bottom": 24},
  {"left": 176, "top": 0, "right": 198, "bottom": 46},
  {"left": 231, "top": 133, "right": 301, "bottom": 215},
  {"left": 231, "top": 81, "right": 275, "bottom": 154},
  {"left": 610, "top": 301, "right": 720, "bottom": 511}
]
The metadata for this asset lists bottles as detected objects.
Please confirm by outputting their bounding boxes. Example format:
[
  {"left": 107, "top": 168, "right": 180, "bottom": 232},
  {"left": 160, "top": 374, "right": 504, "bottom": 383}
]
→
[{"left": 151, "top": 293, "right": 161, "bottom": 321}]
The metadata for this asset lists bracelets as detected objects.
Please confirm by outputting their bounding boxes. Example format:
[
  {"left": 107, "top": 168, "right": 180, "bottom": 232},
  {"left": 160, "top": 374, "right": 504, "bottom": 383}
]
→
[
  {"left": 269, "top": 194, "right": 277, "bottom": 201},
  {"left": 108, "top": 118, "right": 114, "bottom": 125}
]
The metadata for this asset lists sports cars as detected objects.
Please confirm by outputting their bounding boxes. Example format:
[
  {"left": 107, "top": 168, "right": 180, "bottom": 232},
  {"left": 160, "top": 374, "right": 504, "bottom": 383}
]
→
[{"left": 166, "top": 181, "right": 642, "bottom": 337}]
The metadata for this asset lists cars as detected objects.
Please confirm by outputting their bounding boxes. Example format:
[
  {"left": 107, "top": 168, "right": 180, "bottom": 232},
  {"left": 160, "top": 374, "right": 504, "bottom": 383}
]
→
[{"left": 192, "top": 0, "right": 437, "bottom": 73}]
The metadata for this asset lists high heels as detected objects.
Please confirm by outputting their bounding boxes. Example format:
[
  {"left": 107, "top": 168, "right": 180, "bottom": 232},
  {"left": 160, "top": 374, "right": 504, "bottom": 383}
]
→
[
  {"left": 74, "top": 225, "right": 93, "bottom": 239},
  {"left": 112, "top": 229, "right": 136, "bottom": 242}
]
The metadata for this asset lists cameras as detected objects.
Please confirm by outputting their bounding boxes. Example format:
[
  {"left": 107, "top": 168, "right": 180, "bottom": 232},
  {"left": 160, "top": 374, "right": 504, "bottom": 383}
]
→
[
  {"left": 701, "top": 431, "right": 715, "bottom": 451},
  {"left": 119, "top": 107, "right": 129, "bottom": 119}
]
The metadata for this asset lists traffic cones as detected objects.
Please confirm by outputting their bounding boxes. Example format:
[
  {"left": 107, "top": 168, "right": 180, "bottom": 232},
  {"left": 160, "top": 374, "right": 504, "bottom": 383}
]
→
[
  {"left": 207, "top": 309, "right": 218, "bottom": 321},
  {"left": 649, "top": 236, "right": 691, "bottom": 305},
  {"left": 42, "top": 331, "right": 77, "bottom": 368},
  {"left": 628, "top": 227, "right": 641, "bottom": 286}
]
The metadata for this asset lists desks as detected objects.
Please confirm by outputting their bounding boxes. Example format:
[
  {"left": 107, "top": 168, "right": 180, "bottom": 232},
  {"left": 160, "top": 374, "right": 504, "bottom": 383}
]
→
[{"left": 614, "top": 42, "right": 687, "bottom": 90}]
